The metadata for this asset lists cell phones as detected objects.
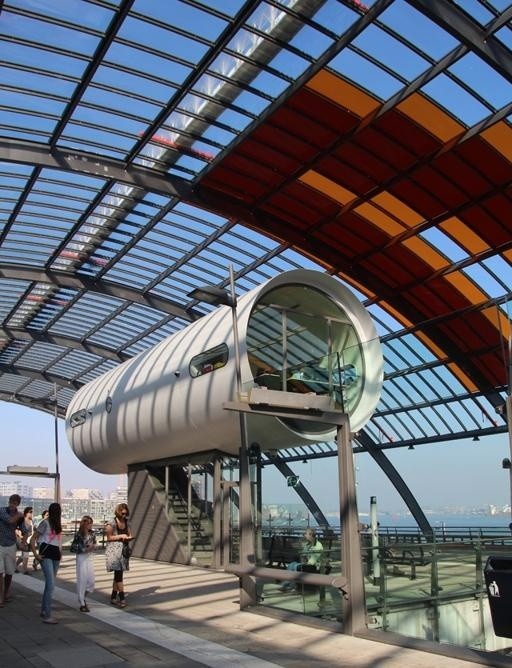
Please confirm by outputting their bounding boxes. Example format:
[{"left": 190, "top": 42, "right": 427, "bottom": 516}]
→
[{"left": 128, "top": 537, "right": 135, "bottom": 539}]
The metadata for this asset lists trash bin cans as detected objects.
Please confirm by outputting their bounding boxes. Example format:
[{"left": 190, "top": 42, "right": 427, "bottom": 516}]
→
[{"left": 484, "top": 556, "right": 511, "bottom": 638}]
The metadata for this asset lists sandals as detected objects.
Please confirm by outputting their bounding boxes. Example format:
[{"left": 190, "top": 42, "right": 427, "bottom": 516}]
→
[
  {"left": 119, "top": 600, "right": 127, "bottom": 607},
  {"left": 80, "top": 606, "right": 89, "bottom": 612},
  {"left": 111, "top": 601, "right": 120, "bottom": 605}
]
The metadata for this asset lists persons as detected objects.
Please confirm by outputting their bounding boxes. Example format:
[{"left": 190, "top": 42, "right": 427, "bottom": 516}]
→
[
  {"left": 0, "top": 494, "right": 27, "bottom": 608},
  {"left": 30, "top": 504, "right": 61, "bottom": 623},
  {"left": 70, "top": 516, "right": 99, "bottom": 613},
  {"left": 34, "top": 510, "right": 49, "bottom": 572},
  {"left": 15, "top": 507, "right": 34, "bottom": 573},
  {"left": 281, "top": 526, "right": 323, "bottom": 590},
  {"left": 106, "top": 504, "right": 134, "bottom": 607}
]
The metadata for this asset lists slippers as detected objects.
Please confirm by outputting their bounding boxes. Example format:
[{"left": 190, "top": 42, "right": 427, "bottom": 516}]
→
[{"left": 40, "top": 616, "right": 60, "bottom": 623}]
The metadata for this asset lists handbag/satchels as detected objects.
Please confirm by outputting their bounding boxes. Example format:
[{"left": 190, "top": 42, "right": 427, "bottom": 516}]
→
[
  {"left": 70, "top": 540, "right": 80, "bottom": 554},
  {"left": 34, "top": 558, "right": 42, "bottom": 569},
  {"left": 122, "top": 545, "right": 132, "bottom": 558},
  {"left": 16, "top": 541, "right": 31, "bottom": 552}
]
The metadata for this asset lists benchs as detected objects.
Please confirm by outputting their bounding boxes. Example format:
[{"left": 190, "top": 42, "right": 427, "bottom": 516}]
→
[{"left": 258, "top": 531, "right": 439, "bottom": 597}]
